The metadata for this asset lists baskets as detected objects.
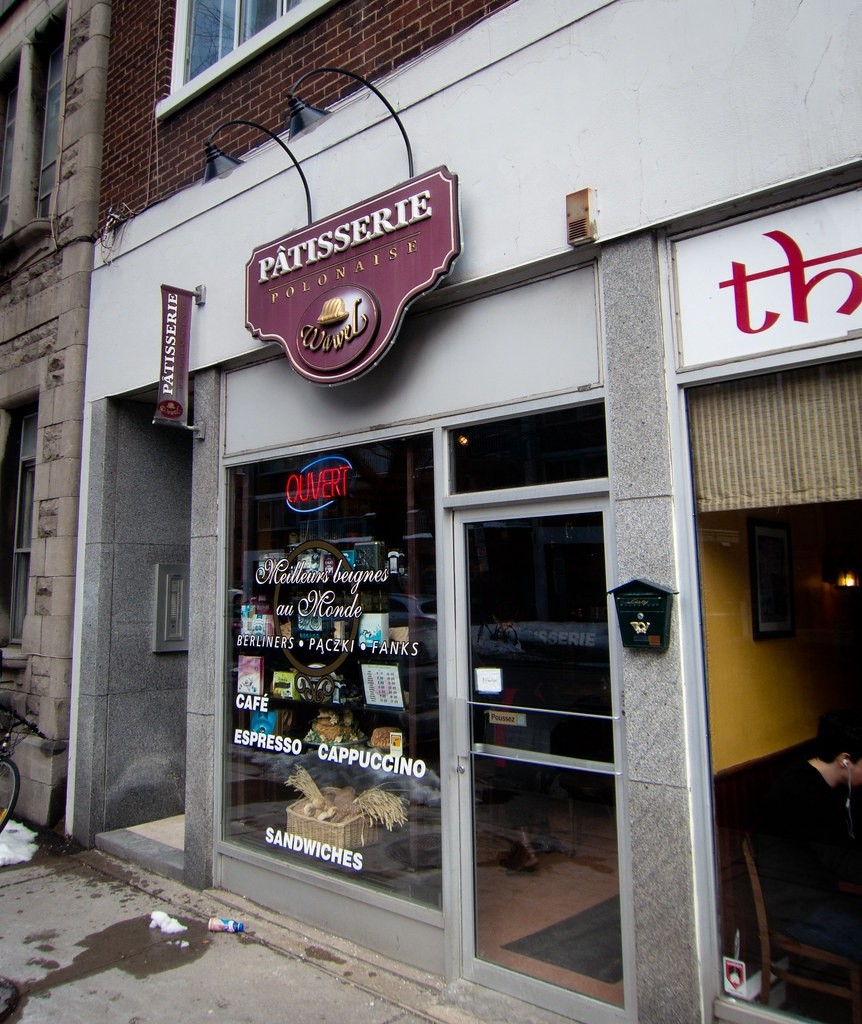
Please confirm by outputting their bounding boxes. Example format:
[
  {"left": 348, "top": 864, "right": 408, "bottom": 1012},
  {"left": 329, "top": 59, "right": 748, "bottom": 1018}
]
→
[{"left": 286, "top": 787, "right": 383, "bottom": 852}]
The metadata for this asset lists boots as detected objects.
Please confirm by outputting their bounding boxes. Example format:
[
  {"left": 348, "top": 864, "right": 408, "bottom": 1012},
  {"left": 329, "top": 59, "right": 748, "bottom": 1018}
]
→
[{"left": 508, "top": 827, "right": 540, "bottom": 871}]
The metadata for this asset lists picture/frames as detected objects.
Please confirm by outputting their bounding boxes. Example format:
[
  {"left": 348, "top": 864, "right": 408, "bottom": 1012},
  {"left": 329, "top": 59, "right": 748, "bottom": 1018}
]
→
[{"left": 746, "top": 515, "right": 797, "bottom": 640}]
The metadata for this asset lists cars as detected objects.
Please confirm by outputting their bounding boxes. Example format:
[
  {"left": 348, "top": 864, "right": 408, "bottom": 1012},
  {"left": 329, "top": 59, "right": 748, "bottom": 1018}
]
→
[{"left": 348, "top": 595, "right": 440, "bottom": 764}]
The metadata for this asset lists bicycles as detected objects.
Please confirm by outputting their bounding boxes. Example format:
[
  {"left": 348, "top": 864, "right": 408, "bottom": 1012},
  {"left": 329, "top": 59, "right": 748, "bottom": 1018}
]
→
[
  {"left": 475, "top": 612, "right": 519, "bottom": 647},
  {"left": 1, "top": 699, "right": 47, "bottom": 837}
]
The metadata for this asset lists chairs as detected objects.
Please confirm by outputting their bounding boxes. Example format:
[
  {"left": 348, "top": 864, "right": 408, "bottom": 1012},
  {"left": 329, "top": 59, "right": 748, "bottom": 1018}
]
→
[{"left": 742, "top": 832, "right": 862, "bottom": 1023}]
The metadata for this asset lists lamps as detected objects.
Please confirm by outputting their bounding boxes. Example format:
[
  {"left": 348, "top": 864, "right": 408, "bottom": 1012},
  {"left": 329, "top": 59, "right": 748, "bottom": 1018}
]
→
[
  {"left": 286, "top": 67, "right": 416, "bottom": 180},
  {"left": 201, "top": 121, "right": 312, "bottom": 227}
]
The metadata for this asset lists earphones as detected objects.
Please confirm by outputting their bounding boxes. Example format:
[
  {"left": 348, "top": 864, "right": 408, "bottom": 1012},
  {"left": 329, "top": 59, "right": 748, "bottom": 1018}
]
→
[{"left": 842, "top": 759, "right": 849, "bottom": 767}]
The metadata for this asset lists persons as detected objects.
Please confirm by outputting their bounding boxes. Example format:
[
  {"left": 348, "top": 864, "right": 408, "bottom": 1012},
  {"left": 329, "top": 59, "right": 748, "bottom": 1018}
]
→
[
  {"left": 750, "top": 698, "right": 861, "bottom": 966},
  {"left": 484, "top": 610, "right": 557, "bottom": 871}
]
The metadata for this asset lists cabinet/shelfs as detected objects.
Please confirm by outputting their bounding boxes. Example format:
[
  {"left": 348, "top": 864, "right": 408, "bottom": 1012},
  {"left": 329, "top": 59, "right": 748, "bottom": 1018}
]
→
[{"left": 237, "top": 445, "right": 437, "bottom": 883}]
